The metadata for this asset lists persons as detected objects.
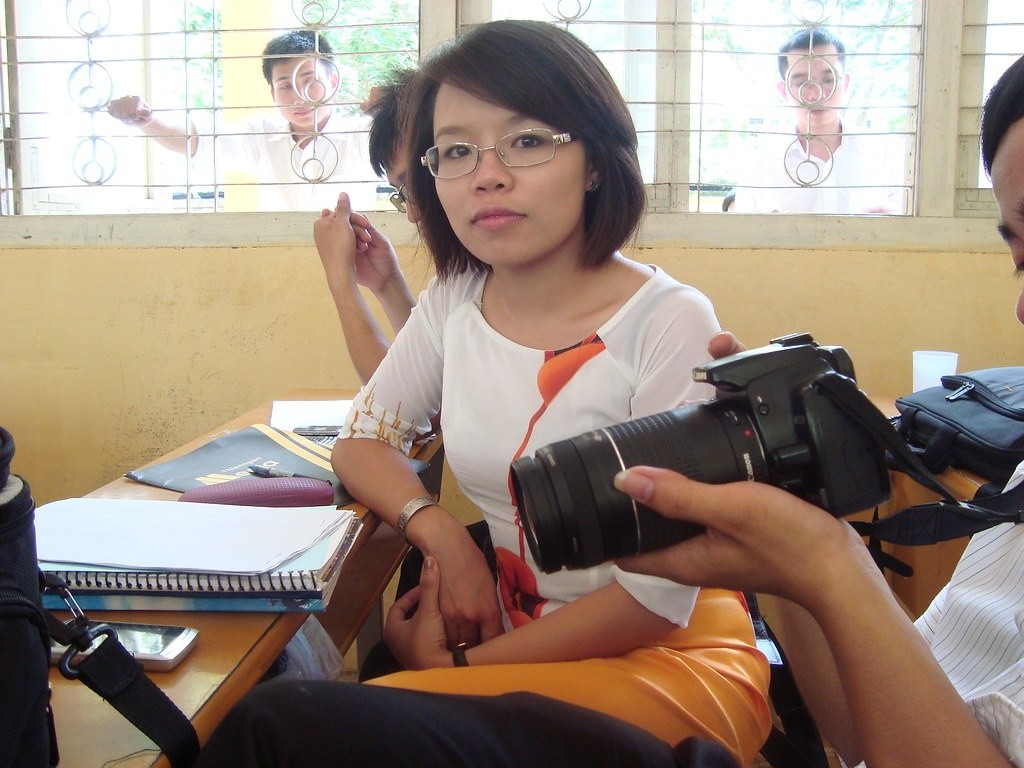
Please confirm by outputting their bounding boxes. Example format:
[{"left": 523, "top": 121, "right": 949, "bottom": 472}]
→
[
  {"left": 614, "top": 52, "right": 1024, "bottom": 768},
  {"left": 192, "top": 18, "right": 774, "bottom": 768},
  {"left": 312, "top": 72, "right": 421, "bottom": 385},
  {"left": 107, "top": 29, "right": 393, "bottom": 210},
  {"left": 721, "top": 26, "right": 907, "bottom": 214}
]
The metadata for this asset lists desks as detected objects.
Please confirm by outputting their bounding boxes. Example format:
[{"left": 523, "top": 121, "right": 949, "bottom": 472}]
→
[
  {"left": 51, "top": 391, "right": 443, "bottom": 768},
  {"left": 845, "top": 397, "right": 992, "bottom": 768}
]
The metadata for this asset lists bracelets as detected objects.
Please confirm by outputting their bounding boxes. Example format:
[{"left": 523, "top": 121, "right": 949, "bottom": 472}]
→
[{"left": 398, "top": 496, "right": 439, "bottom": 531}]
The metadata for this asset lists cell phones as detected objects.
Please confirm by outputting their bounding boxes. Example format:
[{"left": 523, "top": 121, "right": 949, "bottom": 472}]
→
[{"left": 46, "top": 616, "right": 199, "bottom": 674}]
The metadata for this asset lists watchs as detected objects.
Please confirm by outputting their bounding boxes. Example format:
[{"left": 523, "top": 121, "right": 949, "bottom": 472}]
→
[{"left": 452, "top": 642, "right": 471, "bottom": 667}]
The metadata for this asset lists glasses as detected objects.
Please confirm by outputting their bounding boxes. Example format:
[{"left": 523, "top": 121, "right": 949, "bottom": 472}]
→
[
  {"left": 390, "top": 182, "right": 417, "bottom": 214},
  {"left": 420, "top": 128, "right": 580, "bottom": 180}
]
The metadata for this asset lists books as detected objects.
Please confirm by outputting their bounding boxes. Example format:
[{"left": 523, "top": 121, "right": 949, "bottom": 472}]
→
[{"left": 34, "top": 497, "right": 364, "bottom": 613}]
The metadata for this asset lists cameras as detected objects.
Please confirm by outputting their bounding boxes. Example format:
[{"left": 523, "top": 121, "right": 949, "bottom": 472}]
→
[{"left": 509, "top": 328, "right": 894, "bottom": 579}]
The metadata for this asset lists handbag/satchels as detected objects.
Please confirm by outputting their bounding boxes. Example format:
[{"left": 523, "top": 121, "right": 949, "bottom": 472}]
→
[{"left": 894, "top": 366, "right": 1024, "bottom": 479}]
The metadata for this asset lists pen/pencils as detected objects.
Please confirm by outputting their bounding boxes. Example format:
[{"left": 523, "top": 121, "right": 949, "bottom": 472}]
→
[{"left": 248, "top": 464, "right": 333, "bottom": 488}]
